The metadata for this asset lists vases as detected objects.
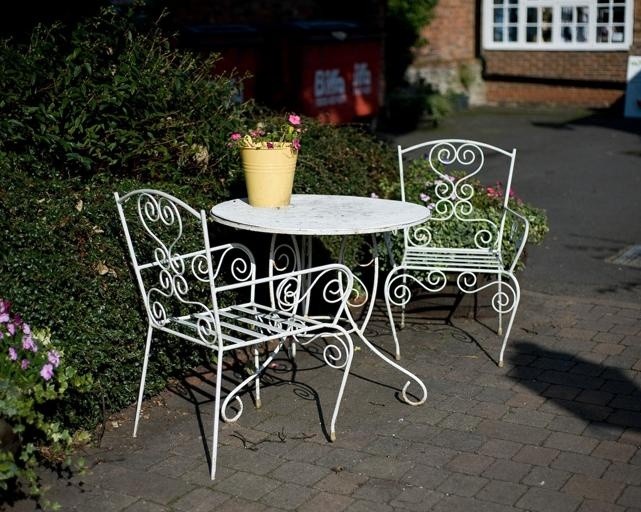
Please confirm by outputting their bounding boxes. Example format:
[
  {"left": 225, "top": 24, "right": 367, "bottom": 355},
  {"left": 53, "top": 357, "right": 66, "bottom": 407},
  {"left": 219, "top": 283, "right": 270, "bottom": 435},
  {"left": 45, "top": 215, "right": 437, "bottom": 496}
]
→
[{"left": 239, "top": 142, "right": 300, "bottom": 207}]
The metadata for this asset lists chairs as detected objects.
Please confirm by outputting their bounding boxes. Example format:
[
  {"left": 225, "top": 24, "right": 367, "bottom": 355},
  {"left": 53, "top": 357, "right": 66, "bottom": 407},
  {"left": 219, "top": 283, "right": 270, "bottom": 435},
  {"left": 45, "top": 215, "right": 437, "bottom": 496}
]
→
[
  {"left": 385, "top": 138, "right": 530, "bottom": 368},
  {"left": 114, "top": 188, "right": 354, "bottom": 479}
]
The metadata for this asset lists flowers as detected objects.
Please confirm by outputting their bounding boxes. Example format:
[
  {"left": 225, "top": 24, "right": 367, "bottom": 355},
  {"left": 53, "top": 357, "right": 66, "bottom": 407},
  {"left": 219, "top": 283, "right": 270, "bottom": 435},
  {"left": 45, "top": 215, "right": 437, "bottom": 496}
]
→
[{"left": 225, "top": 112, "right": 308, "bottom": 156}]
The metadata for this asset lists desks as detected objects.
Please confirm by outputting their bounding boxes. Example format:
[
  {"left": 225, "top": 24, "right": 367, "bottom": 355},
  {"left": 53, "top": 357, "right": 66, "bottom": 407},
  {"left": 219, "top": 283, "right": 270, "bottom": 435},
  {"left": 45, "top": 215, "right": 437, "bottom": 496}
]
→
[{"left": 210, "top": 194, "right": 433, "bottom": 442}]
